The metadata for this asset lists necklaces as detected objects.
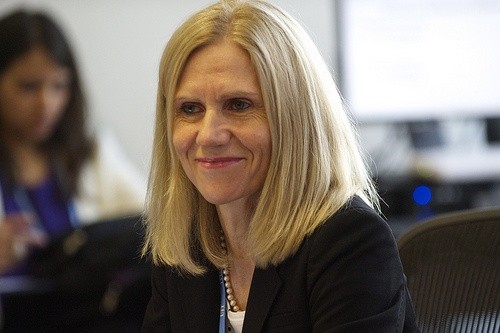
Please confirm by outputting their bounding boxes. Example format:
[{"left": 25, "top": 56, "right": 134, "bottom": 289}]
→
[{"left": 219, "top": 227, "right": 244, "bottom": 314}]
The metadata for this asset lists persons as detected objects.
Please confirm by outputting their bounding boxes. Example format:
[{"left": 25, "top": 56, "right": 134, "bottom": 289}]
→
[
  {"left": 134, "top": 0, "right": 422, "bottom": 332},
  {"left": 1, "top": 10, "right": 99, "bottom": 333}
]
